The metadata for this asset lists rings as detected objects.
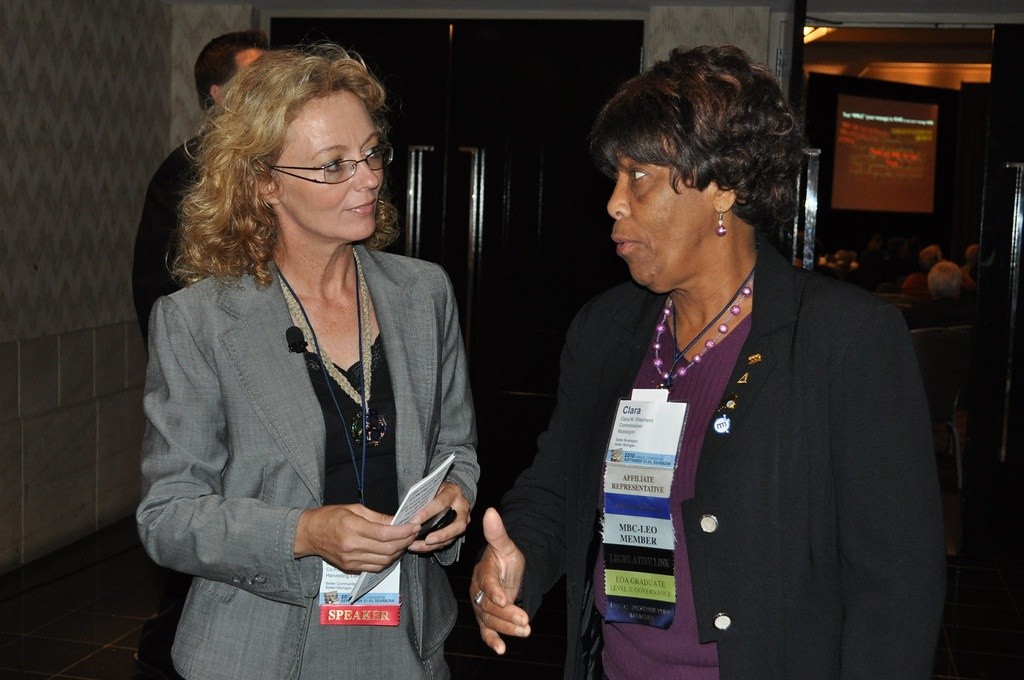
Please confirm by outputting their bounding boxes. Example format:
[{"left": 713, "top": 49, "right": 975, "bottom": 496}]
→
[{"left": 474, "top": 590, "right": 486, "bottom": 607}]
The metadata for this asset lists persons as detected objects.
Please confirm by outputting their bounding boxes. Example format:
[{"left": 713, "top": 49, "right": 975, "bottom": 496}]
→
[
  {"left": 471, "top": 44, "right": 949, "bottom": 680},
  {"left": 130, "top": 28, "right": 481, "bottom": 680}
]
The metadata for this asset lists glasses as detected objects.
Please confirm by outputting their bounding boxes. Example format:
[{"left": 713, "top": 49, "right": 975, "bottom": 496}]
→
[{"left": 265, "top": 141, "right": 393, "bottom": 185}]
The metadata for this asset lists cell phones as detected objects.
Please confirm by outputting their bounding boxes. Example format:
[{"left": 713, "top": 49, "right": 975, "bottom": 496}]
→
[{"left": 415, "top": 507, "right": 457, "bottom": 542}]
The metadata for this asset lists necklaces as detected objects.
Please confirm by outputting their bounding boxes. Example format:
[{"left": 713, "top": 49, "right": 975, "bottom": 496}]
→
[
  {"left": 277, "top": 248, "right": 387, "bottom": 447},
  {"left": 653, "top": 279, "right": 754, "bottom": 380}
]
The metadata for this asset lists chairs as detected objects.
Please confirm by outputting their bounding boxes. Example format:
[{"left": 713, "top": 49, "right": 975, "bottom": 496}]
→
[{"left": 874, "top": 294, "right": 973, "bottom": 492}]
